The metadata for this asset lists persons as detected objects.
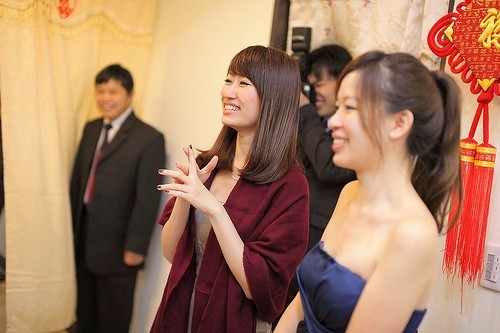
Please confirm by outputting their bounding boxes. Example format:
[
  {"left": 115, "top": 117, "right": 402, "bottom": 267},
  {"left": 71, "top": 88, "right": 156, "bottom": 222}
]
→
[
  {"left": 270, "top": 50, "right": 462, "bottom": 333},
  {"left": 149, "top": 45, "right": 310, "bottom": 333},
  {"left": 297, "top": 45, "right": 358, "bottom": 261},
  {"left": 52, "top": 64, "right": 166, "bottom": 333}
]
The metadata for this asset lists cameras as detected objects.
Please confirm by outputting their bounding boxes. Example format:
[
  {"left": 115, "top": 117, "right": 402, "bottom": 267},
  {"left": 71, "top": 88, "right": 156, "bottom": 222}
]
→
[{"left": 291, "top": 27, "right": 312, "bottom": 102}]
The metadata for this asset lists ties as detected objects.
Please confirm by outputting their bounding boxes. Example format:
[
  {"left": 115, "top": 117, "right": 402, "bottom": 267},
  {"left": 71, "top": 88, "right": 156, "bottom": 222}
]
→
[{"left": 88, "top": 123, "right": 112, "bottom": 204}]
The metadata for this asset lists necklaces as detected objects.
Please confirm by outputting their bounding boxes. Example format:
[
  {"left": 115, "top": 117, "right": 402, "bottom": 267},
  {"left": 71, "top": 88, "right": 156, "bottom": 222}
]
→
[{"left": 230, "top": 171, "right": 239, "bottom": 181}]
